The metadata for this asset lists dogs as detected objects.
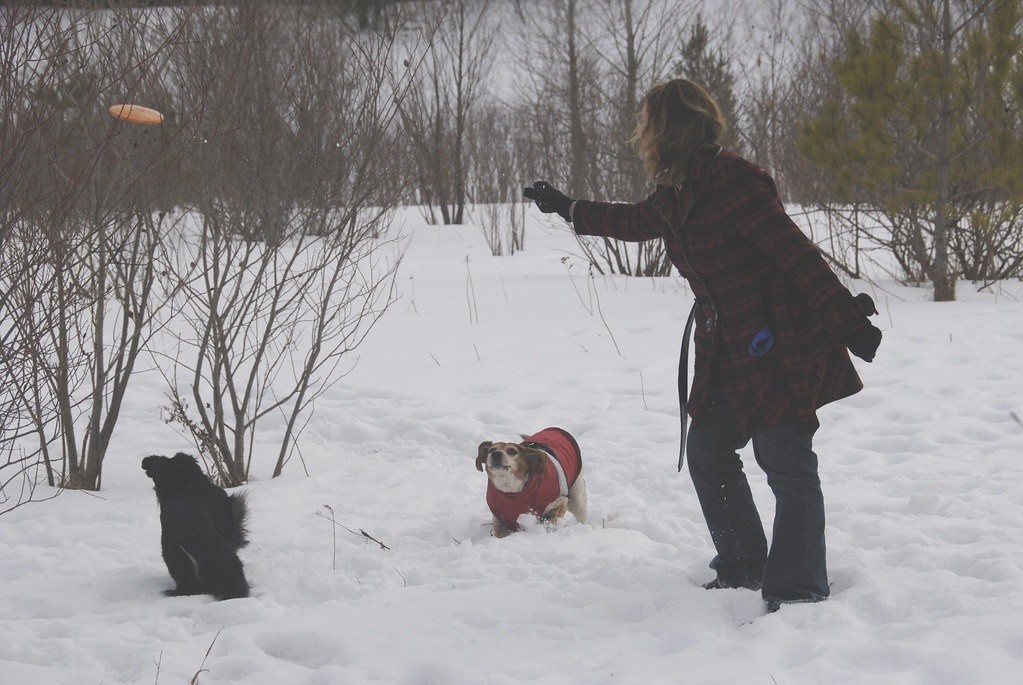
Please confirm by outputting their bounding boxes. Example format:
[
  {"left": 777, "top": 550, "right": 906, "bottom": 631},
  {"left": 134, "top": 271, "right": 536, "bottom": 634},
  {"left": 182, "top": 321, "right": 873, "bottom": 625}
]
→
[
  {"left": 141, "top": 452, "right": 248, "bottom": 602},
  {"left": 475, "top": 427, "right": 588, "bottom": 539}
]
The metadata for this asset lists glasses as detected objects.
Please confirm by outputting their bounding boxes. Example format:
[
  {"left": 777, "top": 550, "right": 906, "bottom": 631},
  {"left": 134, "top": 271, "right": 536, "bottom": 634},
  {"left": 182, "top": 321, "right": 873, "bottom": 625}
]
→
[{"left": 636, "top": 113, "right": 647, "bottom": 127}]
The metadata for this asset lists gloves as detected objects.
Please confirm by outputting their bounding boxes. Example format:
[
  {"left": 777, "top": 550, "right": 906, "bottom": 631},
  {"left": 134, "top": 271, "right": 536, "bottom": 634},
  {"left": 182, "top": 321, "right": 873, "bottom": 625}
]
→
[
  {"left": 524, "top": 181, "right": 576, "bottom": 222},
  {"left": 837, "top": 287, "right": 882, "bottom": 363}
]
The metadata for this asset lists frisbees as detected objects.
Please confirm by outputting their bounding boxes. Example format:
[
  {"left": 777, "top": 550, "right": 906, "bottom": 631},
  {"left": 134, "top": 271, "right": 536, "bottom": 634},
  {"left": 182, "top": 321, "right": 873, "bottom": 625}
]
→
[{"left": 108, "top": 104, "right": 165, "bottom": 125}]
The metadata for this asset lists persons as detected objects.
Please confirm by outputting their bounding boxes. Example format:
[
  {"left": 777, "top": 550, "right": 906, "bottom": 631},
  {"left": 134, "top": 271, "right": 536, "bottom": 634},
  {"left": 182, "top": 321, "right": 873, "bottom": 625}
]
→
[{"left": 523, "top": 79, "right": 882, "bottom": 610}]
